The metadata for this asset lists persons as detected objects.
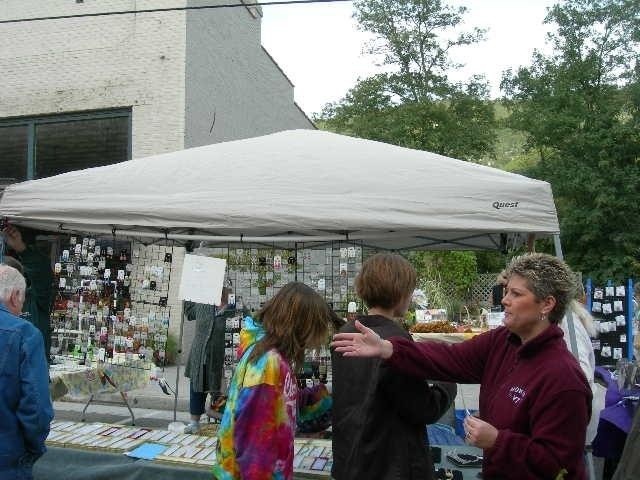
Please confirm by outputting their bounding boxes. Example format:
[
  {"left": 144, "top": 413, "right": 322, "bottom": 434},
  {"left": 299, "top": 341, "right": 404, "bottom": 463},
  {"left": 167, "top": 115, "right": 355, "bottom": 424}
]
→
[
  {"left": 591, "top": 356, "right": 639, "bottom": 478},
  {"left": 330, "top": 252, "right": 457, "bottom": 480},
  {"left": 488, "top": 269, "right": 509, "bottom": 312},
  {"left": 331, "top": 252, "right": 592, "bottom": 480},
  {"left": 185, "top": 286, "right": 253, "bottom": 433},
  {"left": 499, "top": 270, "right": 597, "bottom": 444},
  {"left": 631, "top": 282, "right": 640, "bottom": 361},
  {"left": 1, "top": 263, "right": 53, "bottom": 480},
  {"left": 1, "top": 220, "right": 59, "bottom": 364},
  {"left": 215, "top": 280, "right": 335, "bottom": 479}
]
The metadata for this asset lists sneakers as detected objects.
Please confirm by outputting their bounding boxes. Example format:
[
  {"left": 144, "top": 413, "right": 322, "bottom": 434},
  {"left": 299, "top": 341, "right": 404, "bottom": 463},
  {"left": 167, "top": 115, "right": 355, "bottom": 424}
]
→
[{"left": 184, "top": 424, "right": 199, "bottom": 433}]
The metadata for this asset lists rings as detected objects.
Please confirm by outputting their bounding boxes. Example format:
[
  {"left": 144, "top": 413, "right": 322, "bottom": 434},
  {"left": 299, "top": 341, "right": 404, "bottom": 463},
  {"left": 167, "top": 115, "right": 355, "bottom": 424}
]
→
[{"left": 467, "top": 430, "right": 471, "bottom": 436}]
